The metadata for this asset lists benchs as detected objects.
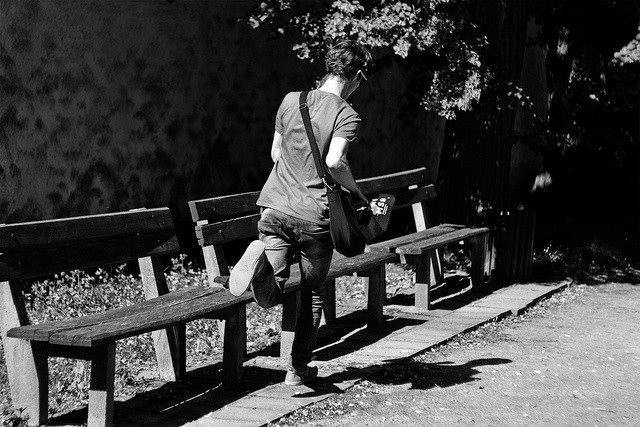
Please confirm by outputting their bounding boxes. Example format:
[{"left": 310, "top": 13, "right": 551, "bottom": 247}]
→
[
  {"left": 0, "top": 205, "right": 254, "bottom": 427},
  {"left": 340, "top": 167, "right": 491, "bottom": 310},
  {"left": 188, "top": 190, "right": 397, "bottom": 357}
]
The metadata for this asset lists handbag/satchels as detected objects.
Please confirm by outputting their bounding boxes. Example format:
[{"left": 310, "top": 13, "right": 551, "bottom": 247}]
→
[{"left": 298, "top": 89, "right": 368, "bottom": 258}]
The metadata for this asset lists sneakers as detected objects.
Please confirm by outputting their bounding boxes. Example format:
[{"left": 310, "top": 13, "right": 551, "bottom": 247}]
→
[
  {"left": 284, "top": 366, "right": 319, "bottom": 386},
  {"left": 228, "top": 239, "right": 266, "bottom": 296}
]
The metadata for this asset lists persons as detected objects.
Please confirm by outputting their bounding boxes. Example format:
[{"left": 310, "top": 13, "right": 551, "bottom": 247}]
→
[{"left": 229, "top": 39, "right": 369, "bottom": 385}]
